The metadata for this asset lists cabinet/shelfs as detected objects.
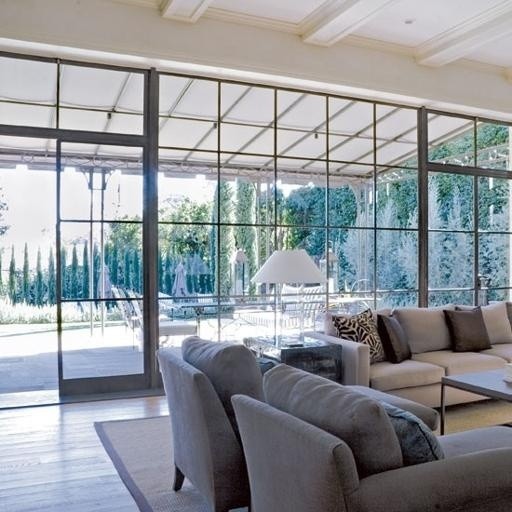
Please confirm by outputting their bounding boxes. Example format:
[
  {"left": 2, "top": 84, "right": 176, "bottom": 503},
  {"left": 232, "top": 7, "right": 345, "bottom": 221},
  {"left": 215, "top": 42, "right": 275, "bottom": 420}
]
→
[{"left": 234, "top": 330, "right": 344, "bottom": 387}]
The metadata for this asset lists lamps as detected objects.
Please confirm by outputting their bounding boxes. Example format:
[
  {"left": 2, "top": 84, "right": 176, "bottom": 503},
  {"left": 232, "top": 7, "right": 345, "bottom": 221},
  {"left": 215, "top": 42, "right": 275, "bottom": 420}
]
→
[{"left": 247, "top": 247, "right": 329, "bottom": 347}]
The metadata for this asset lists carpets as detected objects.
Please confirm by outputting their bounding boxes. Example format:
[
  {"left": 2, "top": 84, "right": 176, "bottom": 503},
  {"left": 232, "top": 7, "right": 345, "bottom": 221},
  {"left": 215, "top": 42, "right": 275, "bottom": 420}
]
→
[{"left": 89, "top": 410, "right": 250, "bottom": 511}]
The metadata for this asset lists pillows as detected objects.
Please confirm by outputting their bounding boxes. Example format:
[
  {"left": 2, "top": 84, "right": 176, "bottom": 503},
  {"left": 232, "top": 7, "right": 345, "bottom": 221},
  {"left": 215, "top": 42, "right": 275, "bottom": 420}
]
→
[
  {"left": 250, "top": 351, "right": 278, "bottom": 378},
  {"left": 328, "top": 299, "right": 511, "bottom": 367},
  {"left": 255, "top": 358, "right": 404, "bottom": 481},
  {"left": 361, "top": 389, "right": 447, "bottom": 469},
  {"left": 172, "top": 328, "right": 271, "bottom": 447}
]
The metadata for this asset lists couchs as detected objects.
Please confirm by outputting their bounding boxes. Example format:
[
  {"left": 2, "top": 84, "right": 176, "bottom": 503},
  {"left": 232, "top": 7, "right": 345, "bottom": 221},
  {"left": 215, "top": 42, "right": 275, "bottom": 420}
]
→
[
  {"left": 223, "top": 360, "right": 509, "bottom": 510},
  {"left": 150, "top": 340, "right": 276, "bottom": 512},
  {"left": 297, "top": 299, "right": 512, "bottom": 414}
]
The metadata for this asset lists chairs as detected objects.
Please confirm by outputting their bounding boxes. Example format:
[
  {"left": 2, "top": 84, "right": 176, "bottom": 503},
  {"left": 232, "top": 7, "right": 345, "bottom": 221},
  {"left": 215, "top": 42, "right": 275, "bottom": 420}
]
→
[{"left": 106, "top": 279, "right": 383, "bottom": 348}]
